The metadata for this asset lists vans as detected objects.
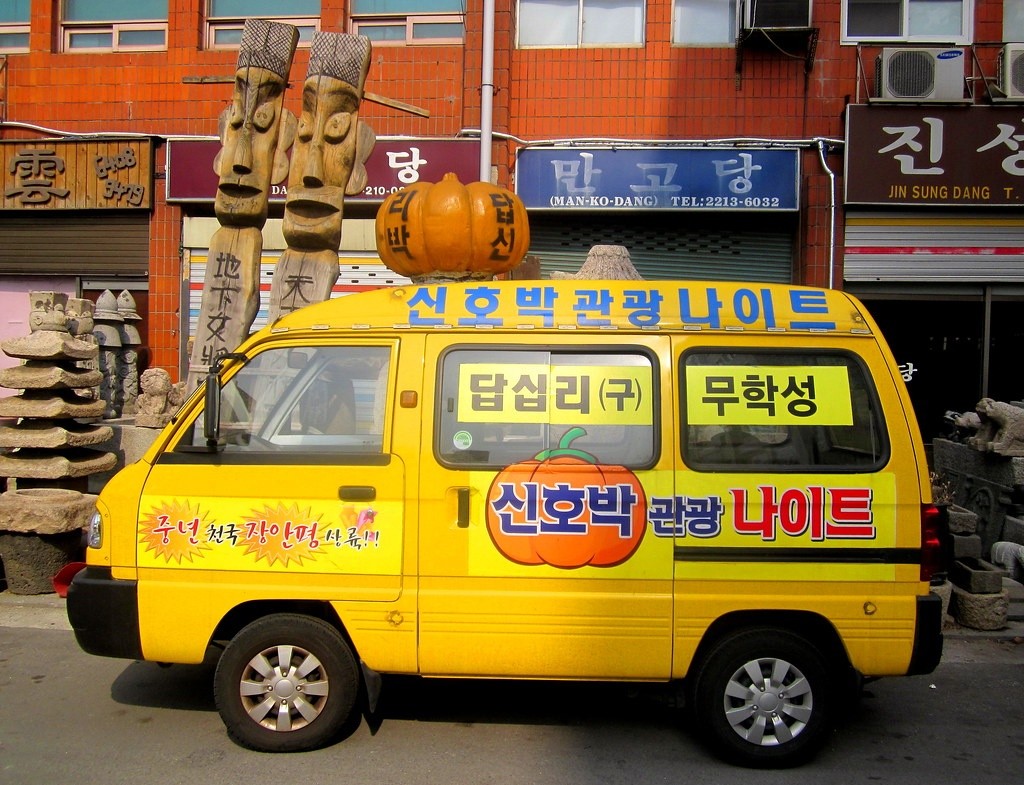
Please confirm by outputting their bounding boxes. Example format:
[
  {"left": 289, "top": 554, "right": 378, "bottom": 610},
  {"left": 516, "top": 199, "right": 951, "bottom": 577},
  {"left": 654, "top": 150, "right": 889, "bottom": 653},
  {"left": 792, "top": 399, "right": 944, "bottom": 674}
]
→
[{"left": 67, "top": 280, "right": 943, "bottom": 768}]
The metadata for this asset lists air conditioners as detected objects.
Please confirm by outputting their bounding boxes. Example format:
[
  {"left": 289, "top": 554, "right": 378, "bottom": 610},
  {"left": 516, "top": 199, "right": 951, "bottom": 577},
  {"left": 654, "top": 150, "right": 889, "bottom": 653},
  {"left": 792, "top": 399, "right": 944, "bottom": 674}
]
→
[
  {"left": 738, "top": 0, "right": 814, "bottom": 29},
  {"left": 878, "top": 46, "right": 968, "bottom": 103},
  {"left": 994, "top": 42, "right": 1024, "bottom": 100}
]
